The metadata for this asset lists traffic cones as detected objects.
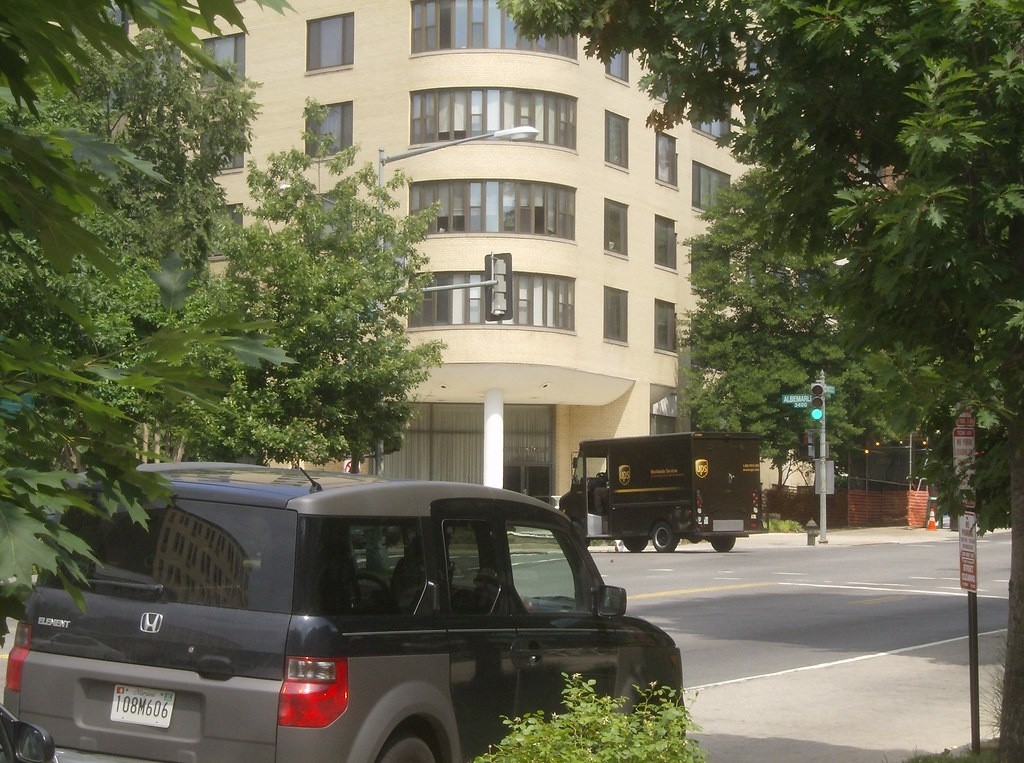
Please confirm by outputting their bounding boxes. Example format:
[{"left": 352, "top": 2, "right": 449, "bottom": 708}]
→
[{"left": 926, "top": 506, "right": 937, "bottom": 531}]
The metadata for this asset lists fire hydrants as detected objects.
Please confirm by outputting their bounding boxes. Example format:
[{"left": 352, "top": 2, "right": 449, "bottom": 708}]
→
[{"left": 806, "top": 517, "right": 820, "bottom": 545}]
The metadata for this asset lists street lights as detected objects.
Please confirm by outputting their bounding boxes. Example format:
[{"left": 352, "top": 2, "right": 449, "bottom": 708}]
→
[{"left": 370, "top": 126, "right": 539, "bottom": 478}]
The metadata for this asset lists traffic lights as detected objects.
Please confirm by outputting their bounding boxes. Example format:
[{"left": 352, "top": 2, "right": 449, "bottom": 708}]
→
[
  {"left": 797, "top": 429, "right": 815, "bottom": 461},
  {"left": 811, "top": 384, "right": 824, "bottom": 421}
]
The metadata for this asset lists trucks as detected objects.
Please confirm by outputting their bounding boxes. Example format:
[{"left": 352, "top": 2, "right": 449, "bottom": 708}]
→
[{"left": 559, "top": 431, "right": 761, "bottom": 553}]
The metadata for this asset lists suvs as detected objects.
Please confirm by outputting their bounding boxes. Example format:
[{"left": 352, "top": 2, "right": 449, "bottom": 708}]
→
[{"left": 3, "top": 462, "right": 686, "bottom": 763}]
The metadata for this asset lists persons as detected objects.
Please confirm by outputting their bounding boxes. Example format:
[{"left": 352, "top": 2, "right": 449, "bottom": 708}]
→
[{"left": 594, "top": 488, "right": 609, "bottom": 516}]
